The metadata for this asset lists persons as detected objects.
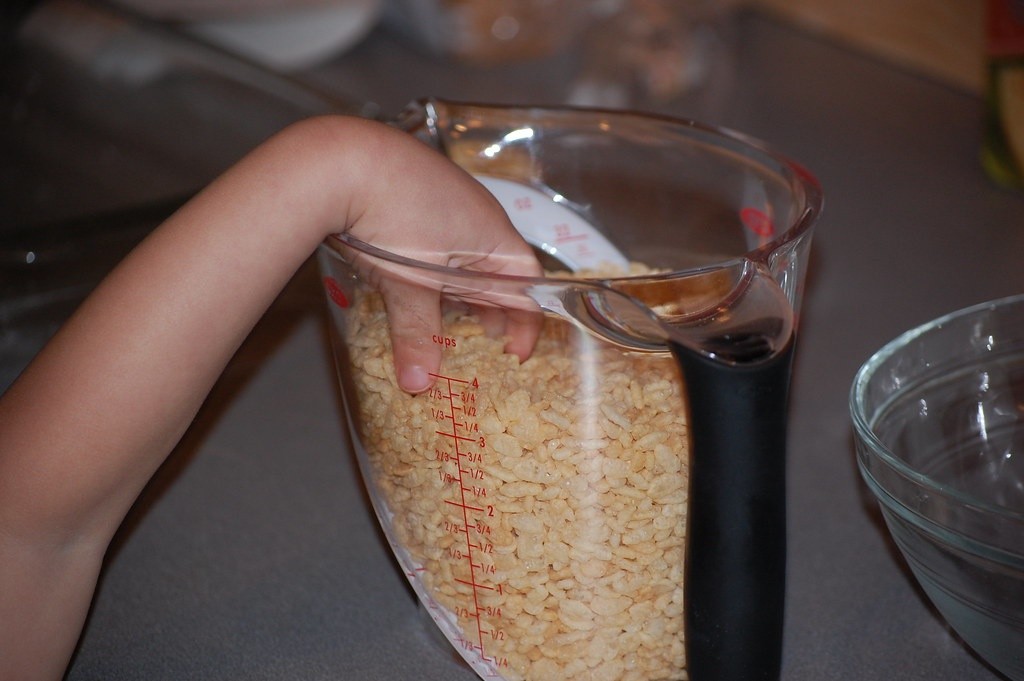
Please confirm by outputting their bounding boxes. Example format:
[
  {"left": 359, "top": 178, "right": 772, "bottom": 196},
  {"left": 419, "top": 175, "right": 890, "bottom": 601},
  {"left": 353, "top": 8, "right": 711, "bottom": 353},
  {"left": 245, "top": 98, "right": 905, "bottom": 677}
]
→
[{"left": 0, "top": 114, "right": 545, "bottom": 681}]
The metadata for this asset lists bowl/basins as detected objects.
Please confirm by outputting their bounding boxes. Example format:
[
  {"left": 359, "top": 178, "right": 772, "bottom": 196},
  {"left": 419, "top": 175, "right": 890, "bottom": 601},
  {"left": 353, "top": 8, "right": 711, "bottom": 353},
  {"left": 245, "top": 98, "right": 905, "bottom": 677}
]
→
[{"left": 849, "top": 296, "right": 1024, "bottom": 681}]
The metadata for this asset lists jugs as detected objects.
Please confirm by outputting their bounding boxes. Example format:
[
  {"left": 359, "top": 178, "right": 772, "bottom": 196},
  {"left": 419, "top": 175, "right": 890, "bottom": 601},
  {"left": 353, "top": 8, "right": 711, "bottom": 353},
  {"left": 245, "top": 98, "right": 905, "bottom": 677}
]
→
[{"left": 310, "top": 92, "right": 822, "bottom": 681}]
